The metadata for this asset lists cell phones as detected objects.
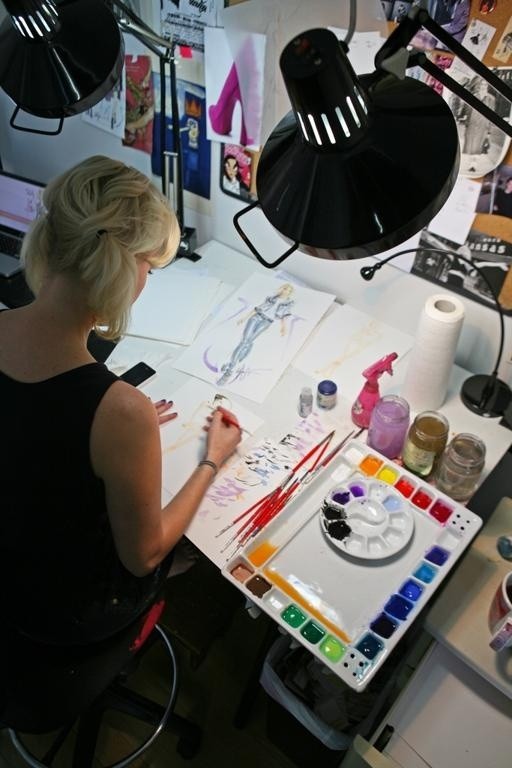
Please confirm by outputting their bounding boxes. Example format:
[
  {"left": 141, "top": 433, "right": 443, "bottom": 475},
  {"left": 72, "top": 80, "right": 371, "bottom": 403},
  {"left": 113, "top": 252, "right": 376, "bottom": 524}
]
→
[{"left": 119, "top": 360, "right": 157, "bottom": 388}]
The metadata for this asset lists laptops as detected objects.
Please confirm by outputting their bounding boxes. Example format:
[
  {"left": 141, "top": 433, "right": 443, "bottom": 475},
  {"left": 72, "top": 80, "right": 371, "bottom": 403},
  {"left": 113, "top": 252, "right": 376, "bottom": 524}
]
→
[{"left": 1, "top": 170, "right": 49, "bottom": 279}]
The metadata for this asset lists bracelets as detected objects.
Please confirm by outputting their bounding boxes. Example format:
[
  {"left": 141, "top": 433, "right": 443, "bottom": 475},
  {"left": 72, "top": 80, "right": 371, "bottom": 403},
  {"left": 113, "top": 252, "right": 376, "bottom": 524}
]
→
[{"left": 198, "top": 460, "right": 218, "bottom": 475}]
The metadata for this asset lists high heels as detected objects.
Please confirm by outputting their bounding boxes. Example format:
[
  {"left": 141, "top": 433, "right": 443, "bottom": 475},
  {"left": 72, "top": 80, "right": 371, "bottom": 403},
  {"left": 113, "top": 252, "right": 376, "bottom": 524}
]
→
[{"left": 209, "top": 61, "right": 253, "bottom": 146}]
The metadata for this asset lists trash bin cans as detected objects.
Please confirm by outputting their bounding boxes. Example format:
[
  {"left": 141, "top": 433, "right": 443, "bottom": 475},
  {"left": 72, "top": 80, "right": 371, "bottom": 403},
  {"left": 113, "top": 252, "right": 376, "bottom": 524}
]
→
[{"left": 259, "top": 633, "right": 376, "bottom": 766}]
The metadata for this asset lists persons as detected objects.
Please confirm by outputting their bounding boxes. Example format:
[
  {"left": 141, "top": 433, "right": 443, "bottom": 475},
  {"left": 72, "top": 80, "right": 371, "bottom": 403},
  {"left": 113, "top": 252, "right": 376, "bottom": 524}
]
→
[
  {"left": 217, "top": 284, "right": 294, "bottom": 386},
  {"left": 0, "top": 155, "right": 241, "bottom": 662},
  {"left": 222, "top": 156, "right": 241, "bottom": 195},
  {"left": 492, "top": 177, "right": 512, "bottom": 219},
  {"left": 464, "top": 79, "right": 496, "bottom": 156}
]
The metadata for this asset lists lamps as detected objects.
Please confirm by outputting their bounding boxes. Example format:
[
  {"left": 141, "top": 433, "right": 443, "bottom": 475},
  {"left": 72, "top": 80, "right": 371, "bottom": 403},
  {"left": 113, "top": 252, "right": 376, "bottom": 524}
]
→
[
  {"left": 230, "top": 5, "right": 511, "bottom": 270},
  {"left": 0, "top": 1, "right": 203, "bottom": 268}
]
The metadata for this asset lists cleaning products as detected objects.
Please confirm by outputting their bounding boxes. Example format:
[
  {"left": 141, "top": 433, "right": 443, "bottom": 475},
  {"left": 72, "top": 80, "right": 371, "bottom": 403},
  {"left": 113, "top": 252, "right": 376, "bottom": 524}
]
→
[{"left": 350, "top": 352, "right": 399, "bottom": 428}]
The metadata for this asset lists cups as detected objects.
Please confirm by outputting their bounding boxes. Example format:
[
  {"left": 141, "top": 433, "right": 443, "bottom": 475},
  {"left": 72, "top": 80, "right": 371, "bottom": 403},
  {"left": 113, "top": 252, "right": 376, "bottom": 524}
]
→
[{"left": 488, "top": 573, "right": 512, "bottom": 652}]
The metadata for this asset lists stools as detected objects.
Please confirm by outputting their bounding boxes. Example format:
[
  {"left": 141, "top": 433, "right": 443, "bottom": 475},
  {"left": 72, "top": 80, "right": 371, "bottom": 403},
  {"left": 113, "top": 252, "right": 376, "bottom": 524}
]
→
[{"left": 1, "top": 579, "right": 210, "bottom": 768}]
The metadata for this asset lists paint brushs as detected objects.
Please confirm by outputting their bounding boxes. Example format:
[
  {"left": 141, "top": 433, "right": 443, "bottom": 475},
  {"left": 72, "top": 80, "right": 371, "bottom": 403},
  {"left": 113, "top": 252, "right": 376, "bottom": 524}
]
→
[
  {"left": 215, "top": 425, "right": 366, "bottom": 562},
  {"left": 208, "top": 401, "right": 253, "bottom": 437}
]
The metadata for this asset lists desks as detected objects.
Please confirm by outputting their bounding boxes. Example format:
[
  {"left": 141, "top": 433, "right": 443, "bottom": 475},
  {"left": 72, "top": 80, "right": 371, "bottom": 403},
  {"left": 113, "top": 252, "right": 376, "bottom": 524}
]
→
[{"left": 103, "top": 237, "right": 511, "bottom": 731}]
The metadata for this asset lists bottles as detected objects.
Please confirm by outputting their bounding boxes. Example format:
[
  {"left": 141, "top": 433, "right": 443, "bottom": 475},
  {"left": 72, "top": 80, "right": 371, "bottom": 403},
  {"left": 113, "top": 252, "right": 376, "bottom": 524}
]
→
[
  {"left": 297, "top": 381, "right": 337, "bottom": 417},
  {"left": 367, "top": 394, "right": 487, "bottom": 502}
]
